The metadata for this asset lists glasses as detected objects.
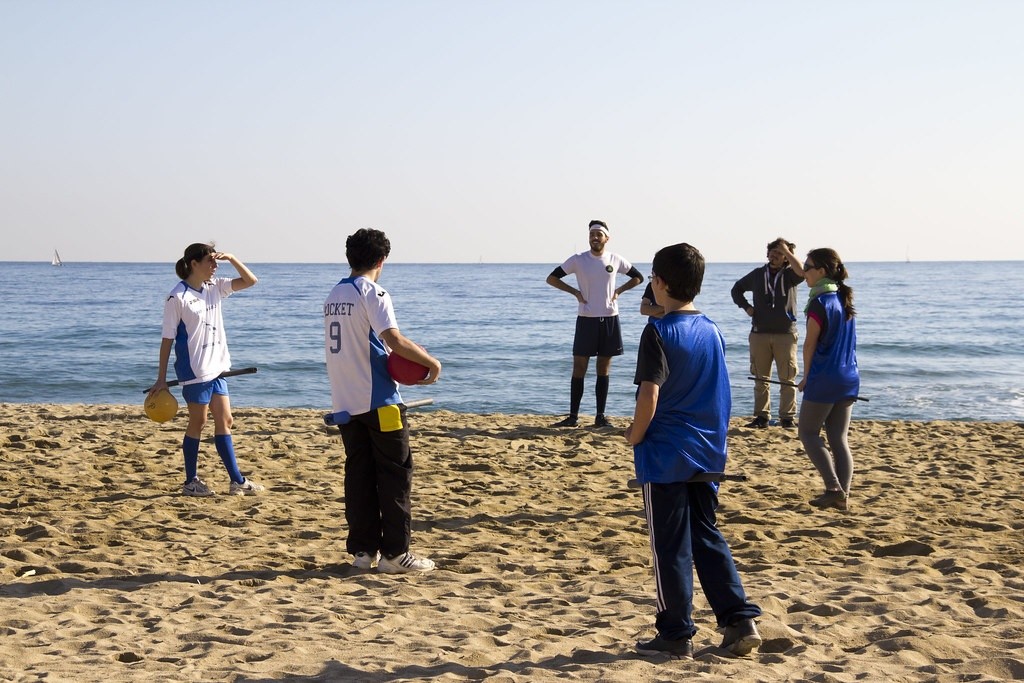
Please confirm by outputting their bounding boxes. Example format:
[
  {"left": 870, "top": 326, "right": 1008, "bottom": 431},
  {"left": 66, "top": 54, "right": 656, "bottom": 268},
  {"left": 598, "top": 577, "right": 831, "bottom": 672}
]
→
[
  {"left": 648, "top": 276, "right": 655, "bottom": 282},
  {"left": 804, "top": 263, "right": 816, "bottom": 271}
]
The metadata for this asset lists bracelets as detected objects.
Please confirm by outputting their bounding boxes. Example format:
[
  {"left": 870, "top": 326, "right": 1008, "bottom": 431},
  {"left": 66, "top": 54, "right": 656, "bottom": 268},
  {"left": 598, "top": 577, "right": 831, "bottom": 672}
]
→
[{"left": 575, "top": 290, "right": 580, "bottom": 295}]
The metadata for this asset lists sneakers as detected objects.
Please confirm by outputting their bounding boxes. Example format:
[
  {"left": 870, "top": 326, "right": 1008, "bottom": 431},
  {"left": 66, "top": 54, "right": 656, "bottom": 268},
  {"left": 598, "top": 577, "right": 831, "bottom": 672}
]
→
[
  {"left": 352, "top": 550, "right": 381, "bottom": 569},
  {"left": 780, "top": 419, "right": 797, "bottom": 428},
  {"left": 555, "top": 417, "right": 578, "bottom": 428},
  {"left": 809, "top": 490, "right": 848, "bottom": 512},
  {"left": 230, "top": 477, "right": 264, "bottom": 496},
  {"left": 635, "top": 633, "right": 694, "bottom": 659},
  {"left": 718, "top": 618, "right": 762, "bottom": 656},
  {"left": 744, "top": 417, "right": 769, "bottom": 427},
  {"left": 595, "top": 414, "right": 613, "bottom": 427},
  {"left": 182, "top": 476, "right": 215, "bottom": 496},
  {"left": 379, "top": 553, "right": 436, "bottom": 574}
]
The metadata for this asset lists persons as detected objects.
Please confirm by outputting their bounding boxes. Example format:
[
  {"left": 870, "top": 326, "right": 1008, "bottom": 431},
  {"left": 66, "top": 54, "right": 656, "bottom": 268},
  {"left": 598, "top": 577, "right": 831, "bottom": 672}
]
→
[
  {"left": 546, "top": 220, "right": 644, "bottom": 429},
  {"left": 323, "top": 229, "right": 442, "bottom": 575},
  {"left": 730, "top": 238, "right": 805, "bottom": 429},
  {"left": 798, "top": 247, "right": 860, "bottom": 510},
  {"left": 151, "top": 243, "right": 265, "bottom": 496},
  {"left": 623, "top": 243, "right": 762, "bottom": 661}
]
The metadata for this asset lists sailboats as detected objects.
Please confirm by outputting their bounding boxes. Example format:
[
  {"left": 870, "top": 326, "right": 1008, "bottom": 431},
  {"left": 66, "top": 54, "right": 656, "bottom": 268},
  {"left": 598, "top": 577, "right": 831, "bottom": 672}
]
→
[{"left": 52, "top": 249, "right": 64, "bottom": 266}]
